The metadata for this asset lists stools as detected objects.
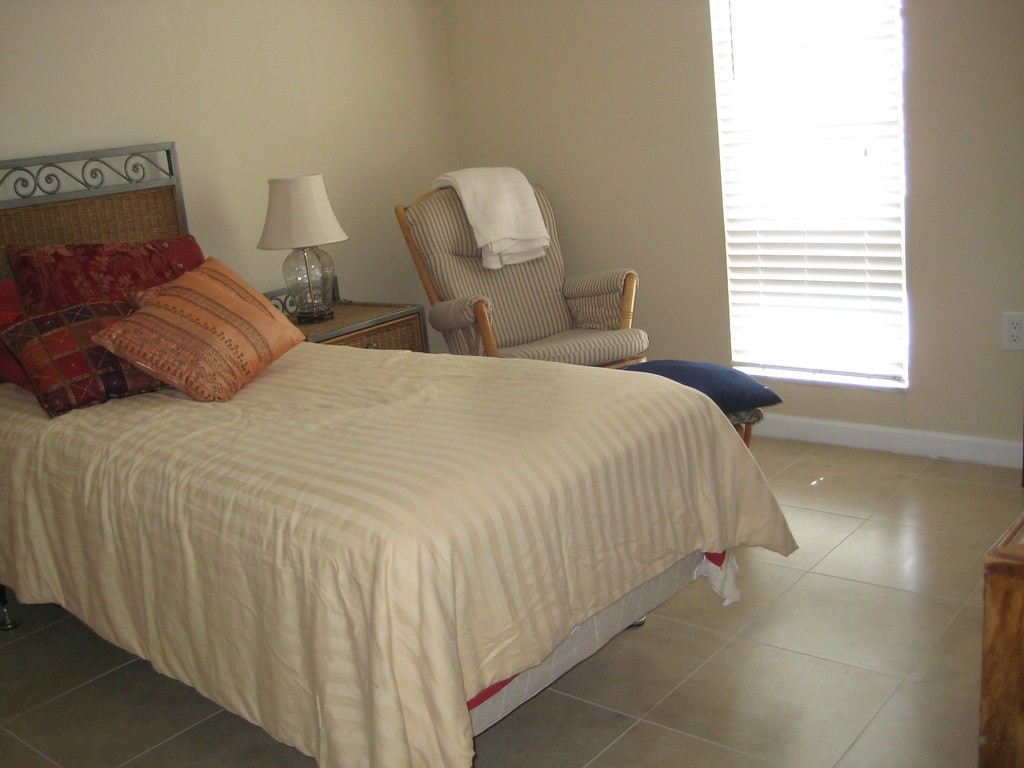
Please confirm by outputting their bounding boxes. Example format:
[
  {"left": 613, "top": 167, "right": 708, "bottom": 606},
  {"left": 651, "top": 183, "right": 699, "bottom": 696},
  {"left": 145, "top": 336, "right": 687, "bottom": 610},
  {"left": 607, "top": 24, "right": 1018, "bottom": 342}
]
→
[{"left": 724, "top": 409, "right": 763, "bottom": 448}]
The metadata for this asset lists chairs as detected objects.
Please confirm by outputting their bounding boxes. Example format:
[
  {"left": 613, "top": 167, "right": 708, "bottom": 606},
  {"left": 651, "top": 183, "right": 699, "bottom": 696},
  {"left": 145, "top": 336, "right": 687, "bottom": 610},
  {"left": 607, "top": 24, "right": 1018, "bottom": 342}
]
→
[{"left": 396, "top": 180, "right": 650, "bottom": 370}]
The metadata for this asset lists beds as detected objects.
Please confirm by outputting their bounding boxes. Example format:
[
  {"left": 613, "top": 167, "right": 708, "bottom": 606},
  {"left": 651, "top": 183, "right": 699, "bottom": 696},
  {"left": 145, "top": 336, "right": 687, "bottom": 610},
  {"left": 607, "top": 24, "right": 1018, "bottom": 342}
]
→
[{"left": 0, "top": 141, "right": 796, "bottom": 768}]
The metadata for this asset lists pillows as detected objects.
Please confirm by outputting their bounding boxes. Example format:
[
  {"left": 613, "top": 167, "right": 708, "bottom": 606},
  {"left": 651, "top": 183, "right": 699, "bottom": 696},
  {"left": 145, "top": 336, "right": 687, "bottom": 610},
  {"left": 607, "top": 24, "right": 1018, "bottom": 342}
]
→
[
  {"left": 0, "top": 293, "right": 176, "bottom": 417},
  {"left": 1, "top": 277, "right": 37, "bottom": 392},
  {"left": 123, "top": 257, "right": 294, "bottom": 325},
  {"left": 7, "top": 237, "right": 178, "bottom": 311},
  {"left": 90, "top": 271, "right": 305, "bottom": 402},
  {"left": 617, "top": 359, "right": 783, "bottom": 414}
]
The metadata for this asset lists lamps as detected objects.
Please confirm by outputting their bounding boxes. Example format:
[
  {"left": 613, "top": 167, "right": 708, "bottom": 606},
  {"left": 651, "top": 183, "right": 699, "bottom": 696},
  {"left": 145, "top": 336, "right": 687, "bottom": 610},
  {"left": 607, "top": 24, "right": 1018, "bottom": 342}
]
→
[{"left": 255, "top": 173, "right": 349, "bottom": 322}]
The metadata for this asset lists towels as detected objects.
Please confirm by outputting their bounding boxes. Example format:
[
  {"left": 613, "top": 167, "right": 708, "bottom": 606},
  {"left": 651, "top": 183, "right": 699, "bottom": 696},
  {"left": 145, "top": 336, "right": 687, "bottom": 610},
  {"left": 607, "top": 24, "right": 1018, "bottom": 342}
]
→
[{"left": 431, "top": 166, "right": 551, "bottom": 270}]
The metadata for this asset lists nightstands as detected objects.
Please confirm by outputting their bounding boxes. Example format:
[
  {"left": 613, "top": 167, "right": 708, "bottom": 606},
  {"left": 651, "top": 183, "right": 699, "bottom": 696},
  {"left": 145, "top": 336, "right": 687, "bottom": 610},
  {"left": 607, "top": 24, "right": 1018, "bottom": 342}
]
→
[{"left": 294, "top": 300, "right": 430, "bottom": 353}]
